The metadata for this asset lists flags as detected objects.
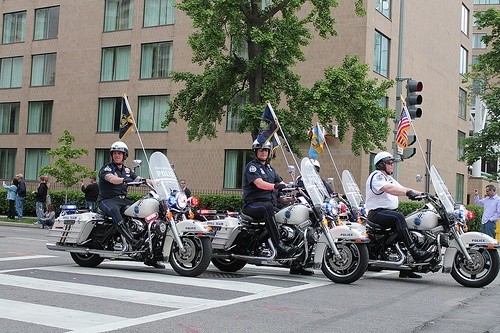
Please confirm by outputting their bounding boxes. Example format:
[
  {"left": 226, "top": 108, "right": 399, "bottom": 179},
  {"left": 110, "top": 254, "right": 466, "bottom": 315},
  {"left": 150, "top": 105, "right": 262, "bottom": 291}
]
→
[
  {"left": 396, "top": 107, "right": 411, "bottom": 149},
  {"left": 269, "top": 135, "right": 280, "bottom": 157},
  {"left": 256, "top": 105, "right": 279, "bottom": 148},
  {"left": 308, "top": 124, "right": 325, "bottom": 159},
  {"left": 118, "top": 97, "right": 134, "bottom": 140}
]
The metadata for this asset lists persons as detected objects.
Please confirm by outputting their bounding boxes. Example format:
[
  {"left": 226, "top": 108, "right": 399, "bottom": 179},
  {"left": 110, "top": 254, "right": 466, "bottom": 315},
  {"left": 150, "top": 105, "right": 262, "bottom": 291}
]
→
[
  {"left": 98, "top": 141, "right": 165, "bottom": 268},
  {"left": 3, "top": 178, "right": 18, "bottom": 219},
  {"left": 179, "top": 180, "right": 191, "bottom": 199},
  {"left": 31, "top": 176, "right": 48, "bottom": 224},
  {"left": 239, "top": 138, "right": 316, "bottom": 276},
  {"left": 16, "top": 174, "right": 26, "bottom": 221},
  {"left": 474, "top": 184, "right": 500, "bottom": 239},
  {"left": 37, "top": 205, "right": 55, "bottom": 229},
  {"left": 365, "top": 151, "right": 440, "bottom": 278},
  {"left": 80, "top": 175, "right": 99, "bottom": 213},
  {"left": 295, "top": 158, "right": 343, "bottom": 200}
]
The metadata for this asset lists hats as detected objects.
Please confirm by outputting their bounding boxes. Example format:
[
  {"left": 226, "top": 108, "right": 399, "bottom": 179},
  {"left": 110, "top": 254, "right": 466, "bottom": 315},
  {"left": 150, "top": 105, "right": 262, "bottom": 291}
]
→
[{"left": 16, "top": 174, "right": 24, "bottom": 179}]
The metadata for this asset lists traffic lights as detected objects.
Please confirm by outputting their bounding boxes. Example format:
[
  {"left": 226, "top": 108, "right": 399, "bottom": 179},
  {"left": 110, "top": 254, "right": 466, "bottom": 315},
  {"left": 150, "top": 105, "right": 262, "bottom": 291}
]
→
[
  {"left": 400, "top": 135, "right": 417, "bottom": 160},
  {"left": 404, "top": 78, "right": 423, "bottom": 119}
]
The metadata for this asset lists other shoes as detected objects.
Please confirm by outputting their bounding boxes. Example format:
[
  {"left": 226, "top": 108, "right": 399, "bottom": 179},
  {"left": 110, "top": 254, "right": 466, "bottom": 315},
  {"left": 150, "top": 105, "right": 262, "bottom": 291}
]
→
[
  {"left": 34, "top": 221, "right": 39, "bottom": 224},
  {"left": 40, "top": 224, "right": 44, "bottom": 228}
]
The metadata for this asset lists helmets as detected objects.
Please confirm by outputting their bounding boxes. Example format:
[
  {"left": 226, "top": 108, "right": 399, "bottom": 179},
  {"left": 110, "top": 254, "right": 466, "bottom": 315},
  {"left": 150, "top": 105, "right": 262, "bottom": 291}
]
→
[
  {"left": 374, "top": 151, "right": 394, "bottom": 166},
  {"left": 252, "top": 139, "right": 271, "bottom": 150},
  {"left": 110, "top": 141, "right": 129, "bottom": 154},
  {"left": 310, "top": 159, "right": 320, "bottom": 168}
]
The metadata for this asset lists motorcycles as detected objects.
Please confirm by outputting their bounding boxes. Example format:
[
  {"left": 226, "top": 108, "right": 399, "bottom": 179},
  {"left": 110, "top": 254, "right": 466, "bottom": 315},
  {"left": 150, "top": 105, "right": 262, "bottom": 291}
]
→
[
  {"left": 196, "top": 157, "right": 371, "bottom": 285},
  {"left": 45, "top": 151, "right": 215, "bottom": 277},
  {"left": 278, "top": 169, "right": 365, "bottom": 225},
  {"left": 343, "top": 165, "right": 500, "bottom": 288}
]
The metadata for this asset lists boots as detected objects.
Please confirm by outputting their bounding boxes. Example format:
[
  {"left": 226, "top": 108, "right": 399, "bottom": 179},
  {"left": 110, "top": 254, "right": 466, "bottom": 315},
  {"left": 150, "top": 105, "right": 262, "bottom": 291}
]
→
[
  {"left": 144, "top": 260, "right": 165, "bottom": 269},
  {"left": 264, "top": 215, "right": 299, "bottom": 259},
  {"left": 399, "top": 269, "right": 422, "bottom": 278},
  {"left": 115, "top": 220, "right": 140, "bottom": 247},
  {"left": 398, "top": 228, "right": 432, "bottom": 263},
  {"left": 289, "top": 262, "right": 314, "bottom": 275}
]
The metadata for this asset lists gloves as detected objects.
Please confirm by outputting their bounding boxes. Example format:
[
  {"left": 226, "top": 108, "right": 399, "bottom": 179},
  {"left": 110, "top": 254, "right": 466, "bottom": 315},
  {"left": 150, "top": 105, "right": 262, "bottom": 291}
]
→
[
  {"left": 421, "top": 192, "right": 427, "bottom": 195},
  {"left": 274, "top": 183, "right": 288, "bottom": 193},
  {"left": 123, "top": 177, "right": 133, "bottom": 186},
  {"left": 406, "top": 190, "right": 422, "bottom": 201}
]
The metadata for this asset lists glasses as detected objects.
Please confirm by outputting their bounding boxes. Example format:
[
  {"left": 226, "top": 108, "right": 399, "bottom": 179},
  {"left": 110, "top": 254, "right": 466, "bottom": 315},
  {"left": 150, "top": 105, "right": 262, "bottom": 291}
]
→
[{"left": 485, "top": 189, "right": 492, "bottom": 192}]
